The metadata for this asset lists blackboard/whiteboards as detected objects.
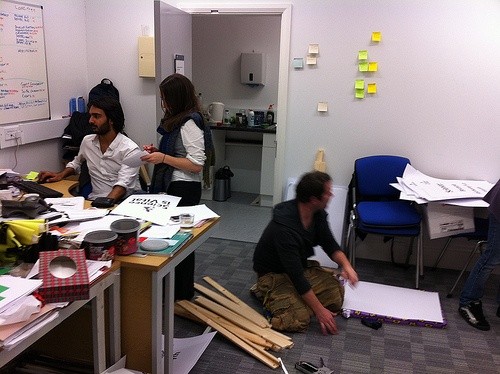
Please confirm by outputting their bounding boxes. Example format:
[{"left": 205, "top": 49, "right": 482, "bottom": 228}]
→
[
  {"left": 284, "top": 178, "right": 348, "bottom": 268},
  {"left": 0, "top": 0, "right": 51, "bottom": 126}
]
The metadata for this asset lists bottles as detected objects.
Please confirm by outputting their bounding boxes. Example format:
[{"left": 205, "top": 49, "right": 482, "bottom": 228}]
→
[
  {"left": 69, "top": 97, "right": 76, "bottom": 116},
  {"left": 248, "top": 109, "right": 254, "bottom": 126},
  {"left": 236, "top": 113, "right": 242, "bottom": 124},
  {"left": 223, "top": 110, "right": 231, "bottom": 124},
  {"left": 266, "top": 104, "right": 274, "bottom": 124},
  {"left": 78, "top": 97, "right": 85, "bottom": 113}
]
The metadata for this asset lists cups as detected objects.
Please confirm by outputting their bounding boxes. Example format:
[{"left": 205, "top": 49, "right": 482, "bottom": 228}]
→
[
  {"left": 84, "top": 231, "right": 118, "bottom": 264},
  {"left": 179, "top": 213, "right": 195, "bottom": 234},
  {"left": 110, "top": 218, "right": 141, "bottom": 255}
]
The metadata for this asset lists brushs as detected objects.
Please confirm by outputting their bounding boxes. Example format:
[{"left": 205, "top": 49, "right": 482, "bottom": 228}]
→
[{"left": 314, "top": 150, "right": 326, "bottom": 172}]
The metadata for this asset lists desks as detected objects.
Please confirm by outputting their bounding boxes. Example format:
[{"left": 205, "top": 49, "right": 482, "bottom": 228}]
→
[{"left": 0, "top": 178, "right": 222, "bottom": 374}]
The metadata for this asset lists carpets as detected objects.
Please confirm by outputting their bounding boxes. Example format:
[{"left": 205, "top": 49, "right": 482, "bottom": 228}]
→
[{"left": 174, "top": 235, "right": 500, "bottom": 374}]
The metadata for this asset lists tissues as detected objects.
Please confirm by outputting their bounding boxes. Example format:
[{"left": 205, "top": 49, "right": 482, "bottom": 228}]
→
[{"left": 37, "top": 247, "right": 90, "bottom": 304}]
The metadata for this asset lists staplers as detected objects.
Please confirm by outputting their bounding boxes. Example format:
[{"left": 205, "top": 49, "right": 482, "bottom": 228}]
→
[{"left": 360, "top": 317, "right": 382, "bottom": 329}]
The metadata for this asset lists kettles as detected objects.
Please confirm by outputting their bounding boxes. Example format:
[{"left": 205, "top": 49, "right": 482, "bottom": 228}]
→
[{"left": 207, "top": 101, "right": 224, "bottom": 123}]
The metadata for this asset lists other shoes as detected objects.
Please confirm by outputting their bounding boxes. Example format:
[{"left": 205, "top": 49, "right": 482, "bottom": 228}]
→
[{"left": 250, "top": 284, "right": 263, "bottom": 297}]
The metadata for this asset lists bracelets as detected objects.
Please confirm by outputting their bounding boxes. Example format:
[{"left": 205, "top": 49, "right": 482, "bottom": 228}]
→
[{"left": 162, "top": 155, "right": 166, "bottom": 163}]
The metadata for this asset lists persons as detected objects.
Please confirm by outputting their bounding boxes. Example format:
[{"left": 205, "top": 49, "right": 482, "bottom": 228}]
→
[
  {"left": 458, "top": 179, "right": 500, "bottom": 332},
  {"left": 249, "top": 171, "right": 358, "bottom": 337},
  {"left": 37, "top": 94, "right": 140, "bottom": 202},
  {"left": 139, "top": 74, "right": 216, "bottom": 302}
]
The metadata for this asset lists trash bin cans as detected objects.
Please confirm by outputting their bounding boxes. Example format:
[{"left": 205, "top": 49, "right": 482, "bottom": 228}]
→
[{"left": 215, "top": 168, "right": 233, "bottom": 202}]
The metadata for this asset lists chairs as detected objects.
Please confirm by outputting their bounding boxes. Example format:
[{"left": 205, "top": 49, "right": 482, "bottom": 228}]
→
[{"left": 346, "top": 155, "right": 424, "bottom": 287}]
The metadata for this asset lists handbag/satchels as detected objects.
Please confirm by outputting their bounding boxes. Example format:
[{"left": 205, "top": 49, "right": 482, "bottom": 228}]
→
[{"left": 87, "top": 79, "right": 119, "bottom": 102}]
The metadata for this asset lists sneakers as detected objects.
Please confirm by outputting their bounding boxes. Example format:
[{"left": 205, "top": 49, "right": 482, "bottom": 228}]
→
[
  {"left": 458, "top": 300, "right": 490, "bottom": 331},
  {"left": 496, "top": 307, "right": 500, "bottom": 317}
]
